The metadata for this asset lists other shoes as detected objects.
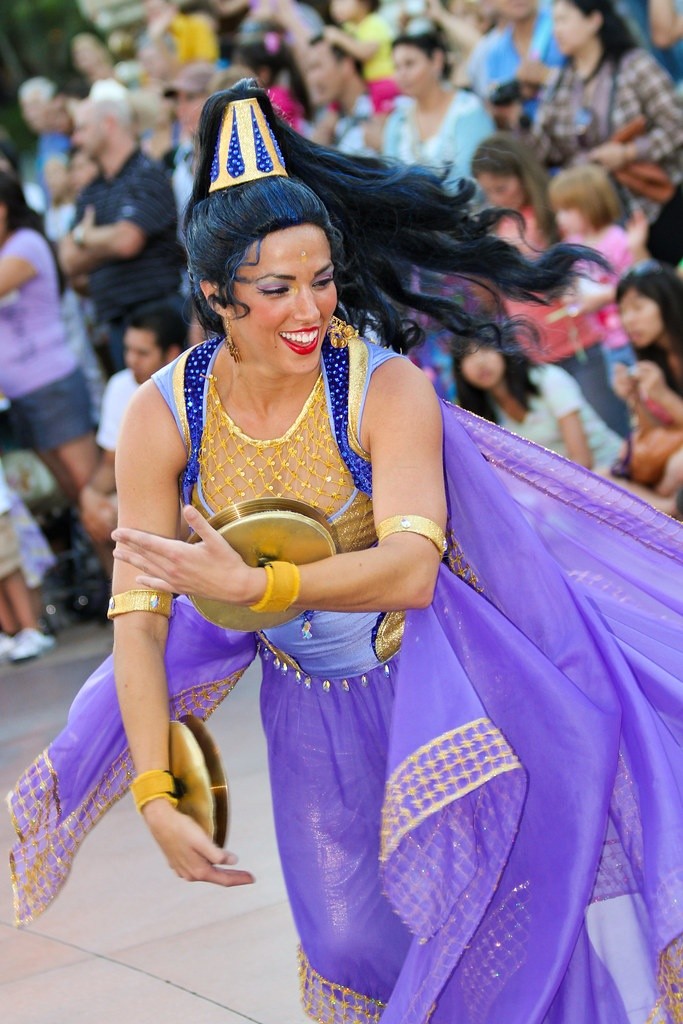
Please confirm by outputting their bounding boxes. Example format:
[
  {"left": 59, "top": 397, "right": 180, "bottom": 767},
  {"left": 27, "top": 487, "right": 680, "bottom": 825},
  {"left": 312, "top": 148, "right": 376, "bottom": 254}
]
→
[{"left": 1, "top": 624, "right": 56, "bottom": 661}]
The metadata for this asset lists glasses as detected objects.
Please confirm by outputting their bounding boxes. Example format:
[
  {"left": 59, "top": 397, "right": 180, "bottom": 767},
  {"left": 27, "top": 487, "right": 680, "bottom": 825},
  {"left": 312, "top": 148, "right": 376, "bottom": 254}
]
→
[{"left": 622, "top": 260, "right": 665, "bottom": 279}]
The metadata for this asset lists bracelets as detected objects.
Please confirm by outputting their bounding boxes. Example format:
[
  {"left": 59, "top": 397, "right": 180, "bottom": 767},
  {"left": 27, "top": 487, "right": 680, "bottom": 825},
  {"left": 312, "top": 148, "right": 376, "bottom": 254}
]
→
[
  {"left": 253, "top": 561, "right": 299, "bottom": 613},
  {"left": 135, "top": 769, "right": 178, "bottom": 814},
  {"left": 76, "top": 229, "right": 87, "bottom": 248}
]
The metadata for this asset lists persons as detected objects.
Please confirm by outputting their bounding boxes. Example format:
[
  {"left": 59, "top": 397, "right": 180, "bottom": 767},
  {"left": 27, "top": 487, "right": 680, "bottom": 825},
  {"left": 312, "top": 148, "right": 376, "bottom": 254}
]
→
[
  {"left": 382, "top": 31, "right": 495, "bottom": 203},
  {"left": 308, "top": 35, "right": 378, "bottom": 159},
  {"left": 58, "top": 80, "right": 191, "bottom": 370},
  {"left": 10, "top": 77, "right": 683, "bottom": 1023},
  {"left": 450, "top": 320, "right": 625, "bottom": 470},
  {"left": 547, "top": 166, "right": 636, "bottom": 390},
  {"left": 0, "top": 0, "right": 683, "bottom": 662}
]
